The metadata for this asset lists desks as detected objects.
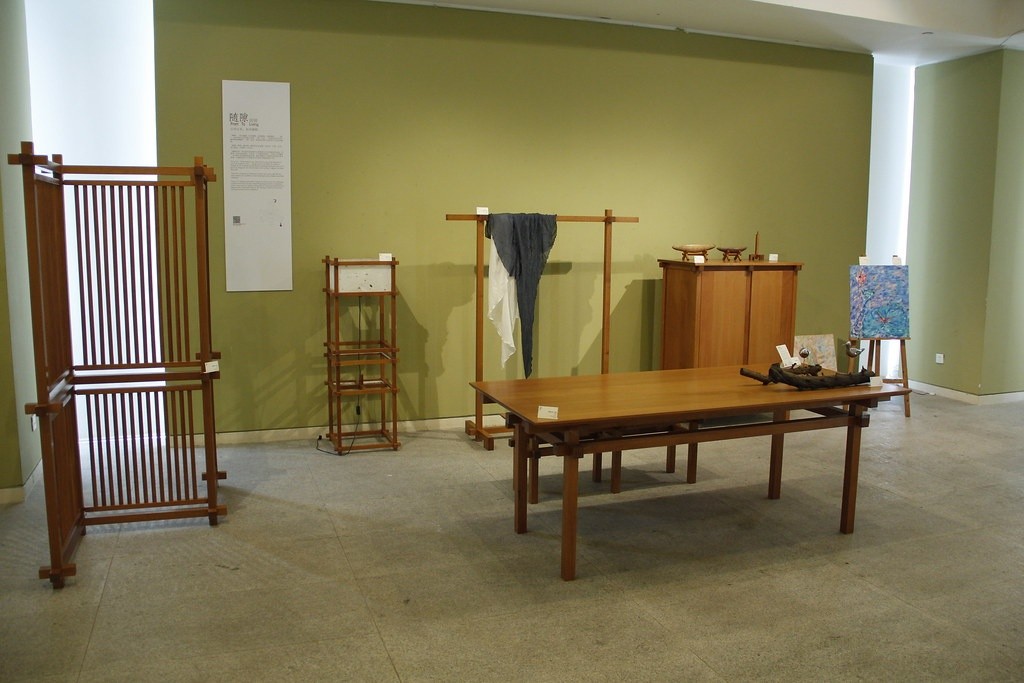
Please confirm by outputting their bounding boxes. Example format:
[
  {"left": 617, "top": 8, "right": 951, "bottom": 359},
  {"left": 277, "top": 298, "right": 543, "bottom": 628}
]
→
[{"left": 470, "top": 361, "right": 914, "bottom": 582}]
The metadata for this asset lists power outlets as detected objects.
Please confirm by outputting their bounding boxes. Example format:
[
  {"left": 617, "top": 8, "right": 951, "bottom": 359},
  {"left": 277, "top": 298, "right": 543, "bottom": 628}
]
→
[{"left": 936, "top": 353, "right": 944, "bottom": 363}]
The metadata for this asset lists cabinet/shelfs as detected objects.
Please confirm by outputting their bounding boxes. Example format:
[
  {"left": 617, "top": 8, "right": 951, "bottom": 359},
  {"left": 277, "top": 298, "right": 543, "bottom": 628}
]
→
[
  {"left": 320, "top": 254, "right": 401, "bottom": 455},
  {"left": 655, "top": 259, "right": 805, "bottom": 432}
]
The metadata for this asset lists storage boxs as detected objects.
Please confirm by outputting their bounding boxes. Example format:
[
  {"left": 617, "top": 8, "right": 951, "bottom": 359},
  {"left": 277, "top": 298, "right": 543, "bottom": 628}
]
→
[{"left": 330, "top": 253, "right": 392, "bottom": 293}]
